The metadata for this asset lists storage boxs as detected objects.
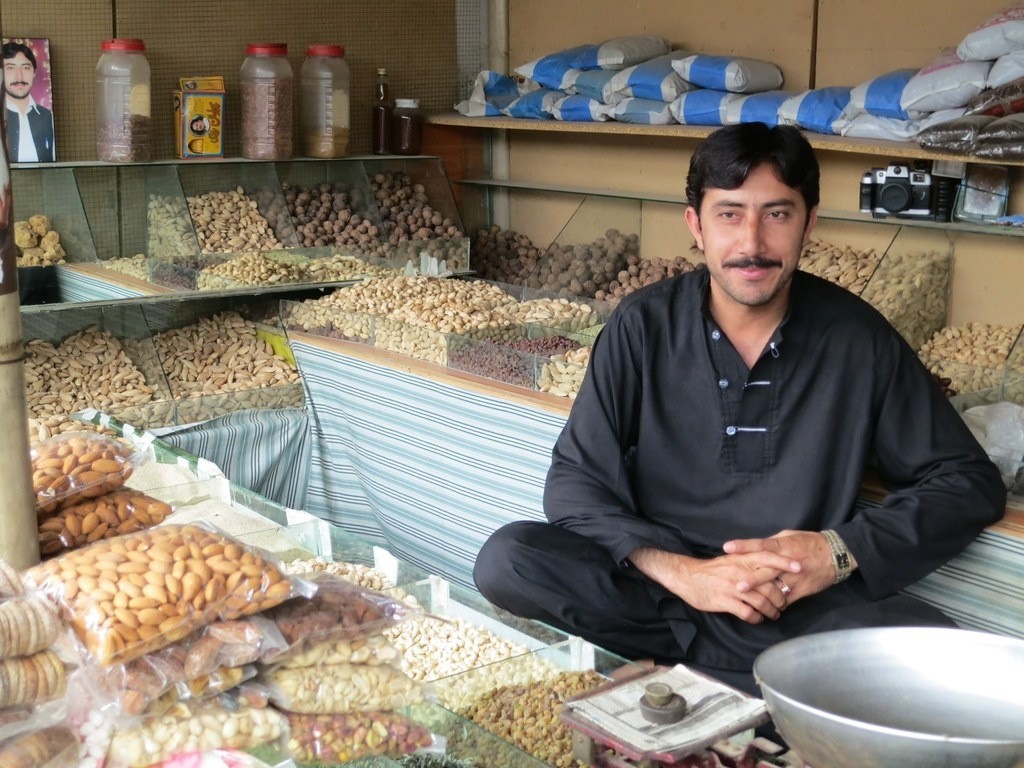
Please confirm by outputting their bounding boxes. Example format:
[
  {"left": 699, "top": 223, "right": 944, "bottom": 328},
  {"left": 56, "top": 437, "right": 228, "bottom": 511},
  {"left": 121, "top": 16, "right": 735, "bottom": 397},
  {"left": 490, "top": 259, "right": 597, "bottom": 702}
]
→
[{"left": 172, "top": 77, "right": 225, "bottom": 160}]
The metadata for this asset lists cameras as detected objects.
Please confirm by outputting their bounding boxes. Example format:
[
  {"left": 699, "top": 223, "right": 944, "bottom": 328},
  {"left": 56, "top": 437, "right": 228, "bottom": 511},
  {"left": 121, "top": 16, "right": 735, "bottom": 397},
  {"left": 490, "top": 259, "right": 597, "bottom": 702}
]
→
[{"left": 858, "top": 161, "right": 953, "bottom": 223}]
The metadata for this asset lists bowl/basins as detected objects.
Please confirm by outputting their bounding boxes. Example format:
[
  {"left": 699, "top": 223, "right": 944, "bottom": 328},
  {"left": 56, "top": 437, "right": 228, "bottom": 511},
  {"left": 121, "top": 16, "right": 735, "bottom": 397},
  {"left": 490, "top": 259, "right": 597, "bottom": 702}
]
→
[{"left": 752, "top": 626, "right": 1024, "bottom": 767}]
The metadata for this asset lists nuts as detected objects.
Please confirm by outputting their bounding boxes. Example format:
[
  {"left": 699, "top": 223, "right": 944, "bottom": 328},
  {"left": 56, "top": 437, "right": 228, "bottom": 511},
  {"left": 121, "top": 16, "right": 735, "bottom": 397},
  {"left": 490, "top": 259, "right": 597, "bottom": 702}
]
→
[
  {"left": 146, "top": 171, "right": 1023, "bottom": 399},
  {"left": 0, "top": 311, "right": 611, "bottom": 768}
]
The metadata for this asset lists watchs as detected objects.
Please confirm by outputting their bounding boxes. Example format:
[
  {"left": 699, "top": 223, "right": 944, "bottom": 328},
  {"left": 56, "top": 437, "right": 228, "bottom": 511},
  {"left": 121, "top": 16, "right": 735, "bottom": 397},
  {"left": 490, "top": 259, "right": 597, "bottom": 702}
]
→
[{"left": 821, "top": 527, "right": 850, "bottom": 584}]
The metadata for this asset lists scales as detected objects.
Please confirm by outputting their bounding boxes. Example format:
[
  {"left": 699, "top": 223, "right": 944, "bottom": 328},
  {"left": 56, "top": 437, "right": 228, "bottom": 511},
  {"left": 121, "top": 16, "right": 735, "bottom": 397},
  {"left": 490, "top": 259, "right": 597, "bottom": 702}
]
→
[{"left": 554, "top": 624, "right": 1024, "bottom": 768}]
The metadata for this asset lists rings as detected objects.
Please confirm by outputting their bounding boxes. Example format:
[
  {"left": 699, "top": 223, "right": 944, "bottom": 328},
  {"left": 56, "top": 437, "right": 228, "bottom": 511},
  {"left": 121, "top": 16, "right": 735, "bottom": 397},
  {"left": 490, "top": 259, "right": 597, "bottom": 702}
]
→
[{"left": 777, "top": 577, "right": 790, "bottom": 595}]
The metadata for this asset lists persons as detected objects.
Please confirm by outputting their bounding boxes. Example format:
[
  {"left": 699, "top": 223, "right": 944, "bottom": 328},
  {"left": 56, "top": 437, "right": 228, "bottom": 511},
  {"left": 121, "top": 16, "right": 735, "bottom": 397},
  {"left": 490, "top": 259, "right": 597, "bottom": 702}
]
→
[
  {"left": 174, "top": 96, "right": 180, "bottom": 111},
  {"left": 190, "top": 117, "right": 209, "bottom": 136},
  {"left": 480, "top": 123, "right": 1009, "bottom": 702},
  {"left": 2, "top": 43, "right": 54, "bottom": 163},
  {"left": 186, "top": 81, "right": 197, "bottom": 90}
]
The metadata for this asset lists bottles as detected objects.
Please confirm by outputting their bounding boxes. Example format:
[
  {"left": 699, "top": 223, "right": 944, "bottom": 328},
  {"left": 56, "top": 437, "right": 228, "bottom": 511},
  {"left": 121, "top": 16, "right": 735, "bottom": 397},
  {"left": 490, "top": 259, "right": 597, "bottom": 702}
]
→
[
  {"left": 94, "top": 38, "right": 152, "bottom": 163},
  {"left": 299, "top": 45, "right": 350, "bottom": 159},
  {"left": 239, "top": 42, "right": 294, "bottom": 160},
  {"left": 372, "top": 68, "right": 392, "bottom": 155},
  {"left": 392, "top": 98, "right": 423, "bottom": 154}
]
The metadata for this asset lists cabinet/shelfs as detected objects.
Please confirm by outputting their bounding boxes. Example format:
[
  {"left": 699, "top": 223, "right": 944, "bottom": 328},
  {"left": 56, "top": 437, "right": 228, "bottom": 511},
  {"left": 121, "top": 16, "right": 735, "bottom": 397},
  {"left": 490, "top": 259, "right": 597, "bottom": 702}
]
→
[{"left": 422, "top": 112, "right": 1024, "bottom": 238}]
